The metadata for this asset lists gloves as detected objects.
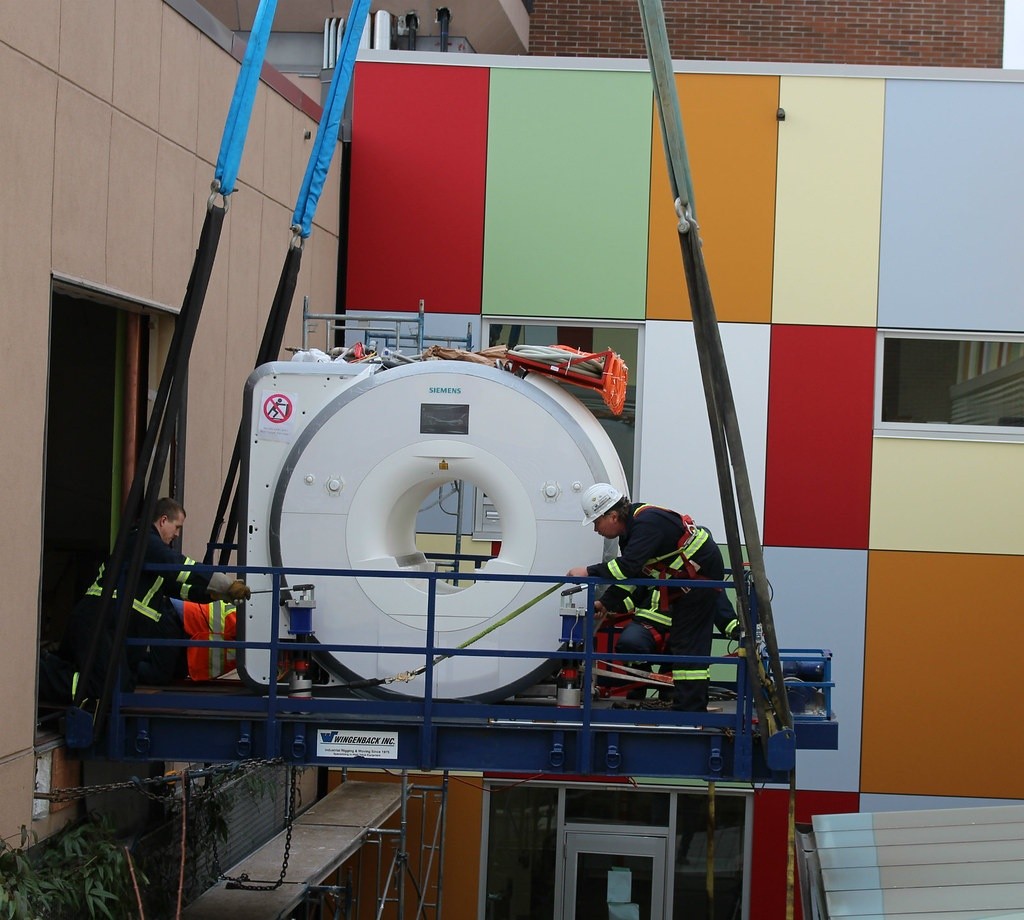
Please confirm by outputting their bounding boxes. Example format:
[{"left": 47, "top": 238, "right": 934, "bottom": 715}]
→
[{"left": 224, "top": 580, "right": 251, "bottom": 606}]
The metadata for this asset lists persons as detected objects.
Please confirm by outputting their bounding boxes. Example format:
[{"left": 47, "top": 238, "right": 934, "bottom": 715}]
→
[
  {"left": 608, "top": 614, "right": 674, "bottom": 704},
  {"left": 565, "top": 486, "right": 742, "bottom": 713},
  {"left": 40, "top": 496, "right": 252, "bottom": 738}
]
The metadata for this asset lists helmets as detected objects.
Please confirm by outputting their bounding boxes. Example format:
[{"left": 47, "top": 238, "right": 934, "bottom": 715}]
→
[{"left": 580, "top": 482, "right": 623, "bottom": 527}]
